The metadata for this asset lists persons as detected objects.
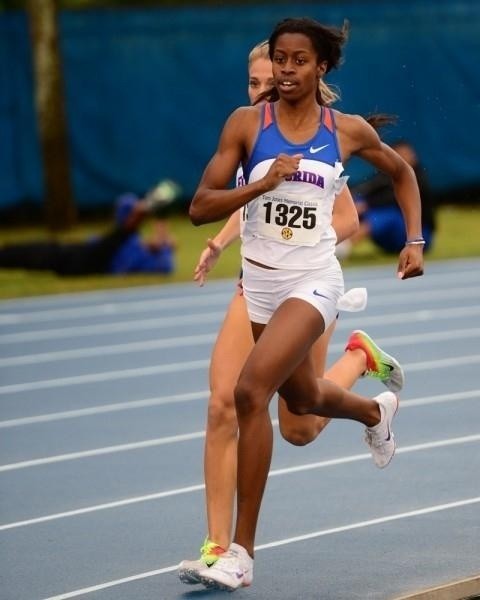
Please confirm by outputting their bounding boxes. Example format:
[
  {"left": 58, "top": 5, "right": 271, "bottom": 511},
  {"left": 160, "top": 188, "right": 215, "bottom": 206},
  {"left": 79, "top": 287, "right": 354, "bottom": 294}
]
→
[
  {"left": 335, "top": 134, "right": 438, "bottom": 262},
  {"left": 186, "top": 14, "right": 428, "bottom": 594},
  {"left": 174, "top": 38, "right": 406, "bottom": 587},
  {"left": 1, "top": 180, "right": 178, "bottom": 279}
]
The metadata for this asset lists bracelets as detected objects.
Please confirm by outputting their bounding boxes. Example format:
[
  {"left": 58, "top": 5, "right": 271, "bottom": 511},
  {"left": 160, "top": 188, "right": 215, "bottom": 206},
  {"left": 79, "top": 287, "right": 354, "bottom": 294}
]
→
[
  {"left": 405, "top": 240, "right": 426, "bottom": 245},
  {"left": 405, "top": 237, "right": 424, "bottom": 244}
]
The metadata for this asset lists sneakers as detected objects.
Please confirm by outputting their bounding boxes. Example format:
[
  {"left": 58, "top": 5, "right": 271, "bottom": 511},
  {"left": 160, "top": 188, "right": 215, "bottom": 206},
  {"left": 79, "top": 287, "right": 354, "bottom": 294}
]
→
[
  {"left": 361, "top": 389, "right": 400, "bottom": 469},
  {"left": 344, "top": 329, "right": 405, "bottom": 393},
  {"left": 177, "top": 536, "right": 230, "bottom": 587},
  {"left": 193, "top": 538, "right": 255, "bottom": 591}
]
